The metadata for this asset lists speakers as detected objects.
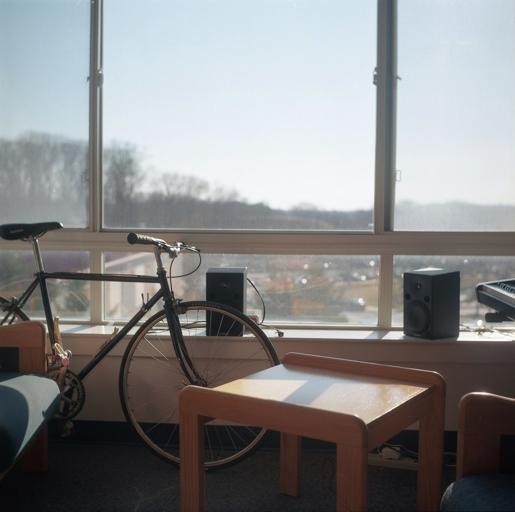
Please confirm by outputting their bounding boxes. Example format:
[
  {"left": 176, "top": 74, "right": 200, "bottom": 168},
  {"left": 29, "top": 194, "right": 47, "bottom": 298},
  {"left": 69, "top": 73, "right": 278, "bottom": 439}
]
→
[
  {"left": 204, "top": 268, "right": 247, "bottom": 337},
  {"left": 403, "top": 266, "right": 462, "bottom": 340}
]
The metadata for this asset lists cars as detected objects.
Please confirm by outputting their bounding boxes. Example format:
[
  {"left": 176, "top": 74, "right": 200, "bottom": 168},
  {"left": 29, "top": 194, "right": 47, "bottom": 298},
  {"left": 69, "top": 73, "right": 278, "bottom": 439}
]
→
[{"left": 0, "top": 222, "right": 278, "bottom": 470}]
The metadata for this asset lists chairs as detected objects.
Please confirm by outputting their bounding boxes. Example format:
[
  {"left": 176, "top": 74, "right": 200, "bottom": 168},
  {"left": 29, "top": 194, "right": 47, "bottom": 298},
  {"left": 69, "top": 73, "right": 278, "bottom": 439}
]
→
[
  {"left": 0, "top": 321, "right": 59, "bottom": 480},
  {"left": 440, "top": 390, "right": 514, "bottom": 512}
]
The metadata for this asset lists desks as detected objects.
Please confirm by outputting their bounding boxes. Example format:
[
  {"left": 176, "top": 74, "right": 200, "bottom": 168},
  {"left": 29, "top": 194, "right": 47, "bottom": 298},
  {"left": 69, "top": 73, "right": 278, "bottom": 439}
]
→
[{"left": 179, "top": 351, "right": 445, "bottom": 511}]
